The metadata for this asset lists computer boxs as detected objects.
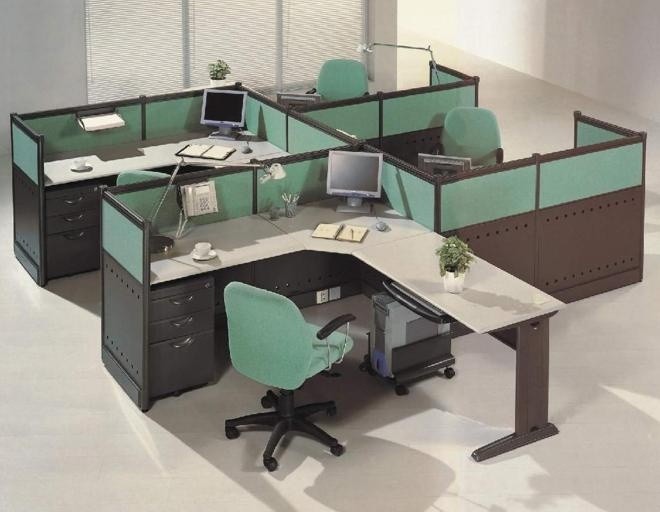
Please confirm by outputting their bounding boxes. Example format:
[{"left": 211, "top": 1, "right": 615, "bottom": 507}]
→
[{"left": 370, "top": 291, "right": 451, "bottom": 379}]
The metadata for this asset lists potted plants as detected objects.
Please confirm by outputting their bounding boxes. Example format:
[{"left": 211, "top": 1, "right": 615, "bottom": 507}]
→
[
  {"left": 206, "top": 58, "right": 234, "bottom": 88},
  {"left": 436, "top": 234, "right": 474, "bottom": 295}
]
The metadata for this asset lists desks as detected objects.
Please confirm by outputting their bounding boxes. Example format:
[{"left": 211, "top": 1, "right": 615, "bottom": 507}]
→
[
  {"left": 44, "top": 127, "right": 290, "bottom": 187},
  {"left": 150, "top": 196, "right": 568, "bottom": 462}
]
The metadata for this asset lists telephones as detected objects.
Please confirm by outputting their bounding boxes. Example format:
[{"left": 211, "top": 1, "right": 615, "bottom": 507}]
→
[{"left": 181, "top": 180, "right": 219, "bottom": 216}]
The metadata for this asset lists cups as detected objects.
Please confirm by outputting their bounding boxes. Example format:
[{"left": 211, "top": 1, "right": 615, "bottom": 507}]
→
[
  {"left": 285, "top": 202, "right": 296, "bottom": 217},
  {"left": 192, "top": 242, "right": 212, "bottom": 256},
  {"left": 269, "top": 207, "right": 280, "bottom": 220},
  {"left": 74, "top": 161, "right": 86, "bottom": 169}
]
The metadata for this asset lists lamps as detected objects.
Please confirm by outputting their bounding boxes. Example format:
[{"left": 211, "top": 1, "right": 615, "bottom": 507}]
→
[
  {"left": 356, "top": 41, "right": 440, "bottom": 86},
  {"left": 76, "top": 113, "right": 127, "bottom": 132},
  {"left": 146, "top": 155, "right": 288, "bottom": 254}
]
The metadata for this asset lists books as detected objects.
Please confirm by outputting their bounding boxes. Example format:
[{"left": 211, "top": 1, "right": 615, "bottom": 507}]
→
[
  {"left": 174, "top": 143, "right": 236, "bottom": 160},
  {"left": 310, "top": 222, "right": 369, "bottom": 243}
]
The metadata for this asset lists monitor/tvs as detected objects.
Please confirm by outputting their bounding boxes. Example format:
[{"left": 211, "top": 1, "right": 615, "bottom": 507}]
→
[
  {"left": 326, "top": 150, "right": 384, "bottom": 214},
  {"left": 418, "top": 153, "right": 471, "bottom": 176},
  {"left": 277, "top": 93, "right": 321, "bottom": 108},
  {"left": 200, "top": 88, "right": 248, "bottom": 141}
]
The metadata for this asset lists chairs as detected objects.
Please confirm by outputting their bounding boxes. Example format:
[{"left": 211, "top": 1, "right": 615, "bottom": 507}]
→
[
  {"left": 305, "top": 58, "right": 369, "bottom": 98},
  {"left": 432, "top": 106, "right": 505, "bottom": 166},
  {"left": 224, "top": 280, "right": 358, "bottom": 472}
]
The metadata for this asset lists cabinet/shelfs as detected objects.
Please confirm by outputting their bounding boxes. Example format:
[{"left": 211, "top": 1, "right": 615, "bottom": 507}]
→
[
  {"left": 149, "top": 274, "right": 217, "bottom": 401},
  {"left": 44, "top": 181, "right": 105, "bottom": 282}
]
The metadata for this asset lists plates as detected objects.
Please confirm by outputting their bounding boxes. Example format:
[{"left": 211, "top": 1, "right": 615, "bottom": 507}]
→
[
  {"left": 190, "top": 250, "right": 217, "bottom": 260},
  {"left": 71, "top": 164, "right": 93, "bottom": 171}
]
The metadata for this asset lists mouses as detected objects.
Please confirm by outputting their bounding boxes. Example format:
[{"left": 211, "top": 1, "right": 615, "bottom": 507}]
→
[
  {"left": 241, "top": 141, "right": 249, "bottom": 153},
  {"left": 376, "top": 222, "right": 386, "bottom": 231}
]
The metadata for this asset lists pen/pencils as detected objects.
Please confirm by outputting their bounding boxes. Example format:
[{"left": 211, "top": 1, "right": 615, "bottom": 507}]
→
[
  {"left": 281, "top": 193, "right": 300, "bottom": 218},
  {"left": 351, "top": 229, "right": 354, "bottom": 239}
]
